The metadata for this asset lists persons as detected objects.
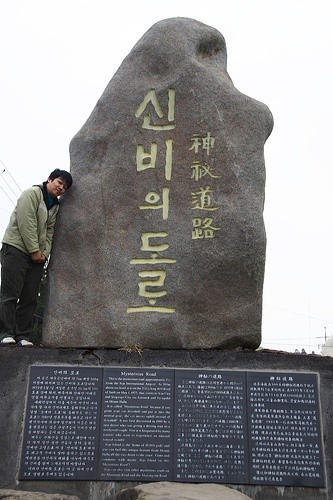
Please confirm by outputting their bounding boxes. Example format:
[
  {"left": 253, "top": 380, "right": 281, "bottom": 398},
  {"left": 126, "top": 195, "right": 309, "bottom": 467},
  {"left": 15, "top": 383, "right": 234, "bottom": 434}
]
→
[{"left": 0, "top": 168, "right": 72, "bottom": 347}]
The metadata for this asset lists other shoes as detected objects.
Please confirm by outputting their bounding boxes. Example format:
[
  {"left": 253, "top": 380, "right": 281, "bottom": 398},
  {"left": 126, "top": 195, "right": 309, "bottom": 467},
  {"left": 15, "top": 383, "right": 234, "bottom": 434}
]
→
[
  {"left": 17, "top": 339, "right": 34, "bottom": 348},
  {"left": 1, "top": 337, "right": 16, "bottom": 344}
]
